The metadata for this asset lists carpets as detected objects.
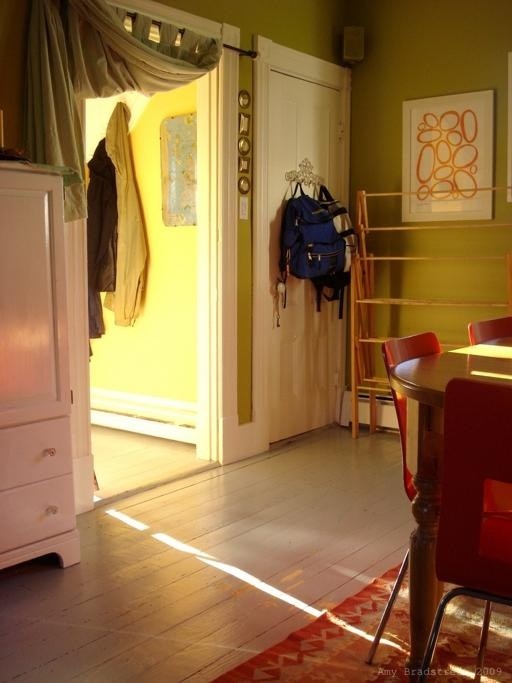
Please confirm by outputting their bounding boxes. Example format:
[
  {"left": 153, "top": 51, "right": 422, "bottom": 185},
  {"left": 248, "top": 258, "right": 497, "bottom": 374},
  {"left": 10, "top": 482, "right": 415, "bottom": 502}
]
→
[{"left": 209, "top": 559, "right": 512, "bottom": 683}]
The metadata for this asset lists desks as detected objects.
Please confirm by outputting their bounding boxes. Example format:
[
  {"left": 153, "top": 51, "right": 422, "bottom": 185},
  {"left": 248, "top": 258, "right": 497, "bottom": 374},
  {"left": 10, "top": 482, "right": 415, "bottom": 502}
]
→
[{"left": 389, "top": 336, "right": 511, "bottom": 683}]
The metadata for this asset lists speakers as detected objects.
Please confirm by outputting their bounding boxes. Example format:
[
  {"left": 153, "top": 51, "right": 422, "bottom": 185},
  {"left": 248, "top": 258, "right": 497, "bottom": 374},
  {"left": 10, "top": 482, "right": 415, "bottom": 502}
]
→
[{"left": 341, "top": 23, "right": 366, "bottom": 66}]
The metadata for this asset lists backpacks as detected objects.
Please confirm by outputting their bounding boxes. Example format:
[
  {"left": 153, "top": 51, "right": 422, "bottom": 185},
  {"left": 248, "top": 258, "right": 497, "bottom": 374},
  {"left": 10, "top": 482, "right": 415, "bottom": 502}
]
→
[{"left": 279, "top": 182, "right": 358, "bottom": 289}]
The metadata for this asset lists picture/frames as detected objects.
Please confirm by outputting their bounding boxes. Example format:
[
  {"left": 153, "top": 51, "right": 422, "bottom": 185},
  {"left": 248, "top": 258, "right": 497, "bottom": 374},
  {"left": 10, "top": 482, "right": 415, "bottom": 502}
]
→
[{"left": 401, "top": 90, "right": 495, "bottom": 222}]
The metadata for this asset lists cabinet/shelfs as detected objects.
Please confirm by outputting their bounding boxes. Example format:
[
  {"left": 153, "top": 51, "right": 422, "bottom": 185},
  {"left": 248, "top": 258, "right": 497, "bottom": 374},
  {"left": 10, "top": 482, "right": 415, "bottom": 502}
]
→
[{"left": 0, "top": 161, "right": 81, "bottom": 570}]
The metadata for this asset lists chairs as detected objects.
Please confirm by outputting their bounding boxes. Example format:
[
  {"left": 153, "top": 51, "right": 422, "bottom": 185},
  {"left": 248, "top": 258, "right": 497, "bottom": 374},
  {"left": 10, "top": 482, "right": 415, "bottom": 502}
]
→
[
  {"left": 366, "top": 332, "right": 442, "bottom": 664},
  {"left": 417, "top": 377, "right": 512, "bottom": 683},
  {"left": 468, "top": 316, "right": 512, "bottom": 345}
]
